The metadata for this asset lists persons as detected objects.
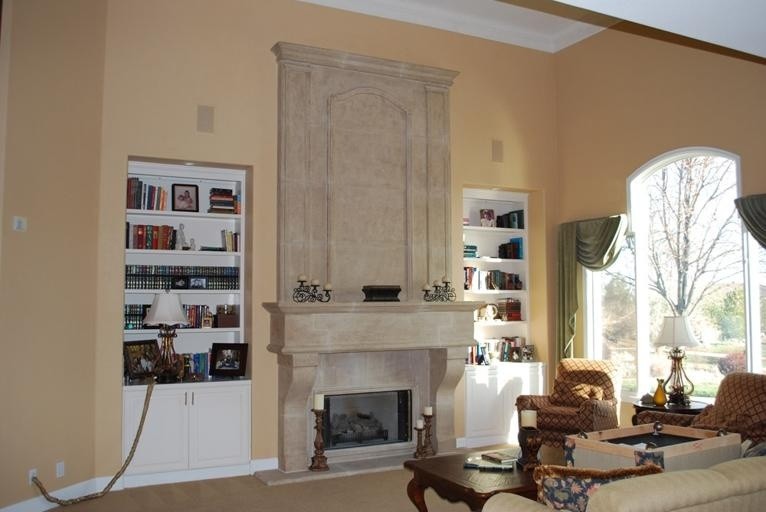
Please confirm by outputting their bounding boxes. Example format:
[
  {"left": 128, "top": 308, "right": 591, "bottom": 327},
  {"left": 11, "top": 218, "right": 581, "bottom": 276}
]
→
[{"left": 481, "top": 210, "right": 495, "bottom": 227}]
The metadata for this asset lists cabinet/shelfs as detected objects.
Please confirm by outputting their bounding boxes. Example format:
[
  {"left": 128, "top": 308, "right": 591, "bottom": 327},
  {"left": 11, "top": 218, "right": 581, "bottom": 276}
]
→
[
  {"left": 462, "top": 188, "right": 531, "bottom": 359},
  {"left": 455, "top": 362, "right": 544, "bottom": 448},
  {"left": 121, "top": 154, "right": 253, "bottom": 384},
  {"left": 117, "top": 379, "right": 253, "bottom": 477}
]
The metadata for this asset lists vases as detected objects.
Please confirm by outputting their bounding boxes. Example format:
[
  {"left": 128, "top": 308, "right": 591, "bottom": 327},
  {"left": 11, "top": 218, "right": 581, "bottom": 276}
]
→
[{"left": 652, "top": 378, "right": 667, "bottom": 407}]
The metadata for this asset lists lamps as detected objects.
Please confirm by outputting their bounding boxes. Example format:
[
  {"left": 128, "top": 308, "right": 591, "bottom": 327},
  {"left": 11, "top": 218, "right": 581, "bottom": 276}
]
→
[
  {"left": 142, "top": 291, "right": 191, "bottom": 382},
  {"left": 652, "top": 315, "right": 696, "bottom": 406}
]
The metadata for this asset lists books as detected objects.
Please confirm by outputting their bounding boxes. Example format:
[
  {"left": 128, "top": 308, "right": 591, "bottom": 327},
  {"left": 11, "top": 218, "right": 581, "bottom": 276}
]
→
[
  {"left": 482, "top": 451, "right": 518, "bottom": 463},
  {"left": 479, "top": 460, "right": 514, "bottom": 472},
  {"left": 463, "top": 455, "right": 484, "bottom": 468},
  {"left": 463, "top": 238, "right": 536, "bottom": 364},
  {"left": 497, "top": 209, "right": 524, "bottom": 229},
  {"left": 175, "top": 353, "right": 211, "bottom": 381},
  {"left": 127, "top": 176, "right": 242, "bottom": 329}
]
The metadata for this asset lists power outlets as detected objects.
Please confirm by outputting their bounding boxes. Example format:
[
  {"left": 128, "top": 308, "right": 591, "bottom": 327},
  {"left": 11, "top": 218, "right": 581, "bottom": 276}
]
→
[{"left": 27, "top": 466, "right": 38, "bottom": 486}]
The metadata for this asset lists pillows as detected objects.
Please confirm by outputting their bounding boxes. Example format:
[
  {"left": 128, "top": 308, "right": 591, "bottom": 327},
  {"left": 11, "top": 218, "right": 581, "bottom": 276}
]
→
[
  {"left": 631, "top": 442, "right": 766, "bottom": 472},
  {"left": 532, "top": 463, "right": 665, "bottom": 511},
  {"left": 689, "top": 404, "right": 753, "bottom": 444},
  {"left": 549, "top": 376, "right": 604, "bottom": 408}
]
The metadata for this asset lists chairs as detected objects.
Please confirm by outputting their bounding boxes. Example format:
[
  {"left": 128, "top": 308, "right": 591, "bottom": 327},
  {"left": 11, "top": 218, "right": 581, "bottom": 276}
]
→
[
  {"left": 631, "top": 370, "right": 766, "bottom": 449},
  {"left": 478, "top": 439, "right": 766, "bottom": 511},
  {"left": 515, "top": 356, "right": 619, "bottom": 465}
]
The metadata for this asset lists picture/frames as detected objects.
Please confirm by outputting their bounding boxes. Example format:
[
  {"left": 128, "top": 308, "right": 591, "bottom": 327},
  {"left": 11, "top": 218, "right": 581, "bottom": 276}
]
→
[
  {"left": 206, "top": 342, "right": 249, "bottom": 378},
  {"left": 171, "top": 182, "right": 200, "bottom": 212},
  {"left": 122, "top": 338, "right": 165, "bottom": 380}
]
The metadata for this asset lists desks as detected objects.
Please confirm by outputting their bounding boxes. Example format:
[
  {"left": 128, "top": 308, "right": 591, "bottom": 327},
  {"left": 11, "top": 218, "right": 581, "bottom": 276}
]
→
[
  {"left": 631, "top": 399, "right": 708, "bottom": 425},
  {"left": 565, "top": 420, "right": 743, "bottom": 471}
]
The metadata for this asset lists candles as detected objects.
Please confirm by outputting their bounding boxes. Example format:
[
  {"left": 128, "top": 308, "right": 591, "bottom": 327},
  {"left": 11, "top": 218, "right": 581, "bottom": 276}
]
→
[
  {"left": 423, "top": 405, "right": 432, "bottom": 416},
  {"left": 441, "top": 275, "right": 452, "bottom": 283},
  {"left": 313, "top": 391, "right": 325, "bottom": 411},
  {"left": 432, "top": 280, "right": 442, "bottom": 287},
  {"left": 311, "top": 279, "right": 320, "bottom": 285},
  {"left": 323, "top": 283, "right": 333, "bottom": 291},
  {"left": 297, "top": 273, "right": 307, "bottom": 282},
  {"left": 415, "top": 417, "right": 424, "bottom": 430},
  {"left": 422, "top": 283, "right": 430, "bottom": 289},
  {"left": 519, "top": 409, "right": 538, "bottom": 431}
]
